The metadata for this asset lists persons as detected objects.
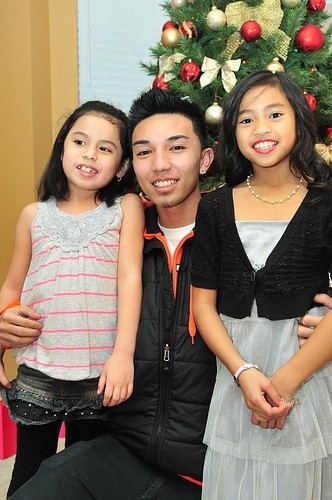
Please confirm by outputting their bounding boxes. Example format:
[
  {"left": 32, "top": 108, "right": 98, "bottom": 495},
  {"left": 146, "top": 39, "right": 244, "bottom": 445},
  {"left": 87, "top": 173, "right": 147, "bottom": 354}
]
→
[{"left": 0, "top": 69, "right": 332, "bottom": 499}]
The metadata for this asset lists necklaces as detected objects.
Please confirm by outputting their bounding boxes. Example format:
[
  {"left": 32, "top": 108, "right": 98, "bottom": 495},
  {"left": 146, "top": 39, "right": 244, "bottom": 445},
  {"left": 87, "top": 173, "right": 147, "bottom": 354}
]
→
[{"left": 246, "top": 171, "right": 306, "bottom": 206}]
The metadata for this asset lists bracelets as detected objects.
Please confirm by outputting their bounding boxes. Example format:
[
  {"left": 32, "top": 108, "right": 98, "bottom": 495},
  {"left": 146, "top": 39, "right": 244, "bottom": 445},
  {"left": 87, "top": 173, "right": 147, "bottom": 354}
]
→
[{"left": 232, "top": 363, "right": 262, "bottom": 388}]
